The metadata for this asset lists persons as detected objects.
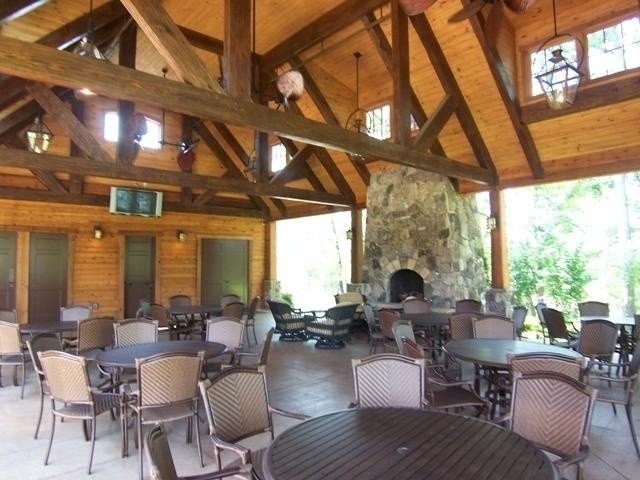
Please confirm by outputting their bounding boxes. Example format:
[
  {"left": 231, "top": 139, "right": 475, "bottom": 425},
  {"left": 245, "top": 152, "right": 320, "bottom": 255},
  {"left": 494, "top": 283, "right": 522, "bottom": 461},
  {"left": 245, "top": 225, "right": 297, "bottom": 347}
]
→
[{"left": 399, "top": 289, "right": 423, "bottom": 302}]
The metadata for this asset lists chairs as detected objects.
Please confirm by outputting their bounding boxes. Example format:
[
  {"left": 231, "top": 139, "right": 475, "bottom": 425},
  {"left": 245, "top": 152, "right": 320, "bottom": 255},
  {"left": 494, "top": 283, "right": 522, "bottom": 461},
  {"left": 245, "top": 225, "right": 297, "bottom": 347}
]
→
[
  {"left": 335, "top": 293, "right": 369, "bottom": 333},
  {"left": 26, "top": 331, "right": 114, "bottom": 440},
  {"left": 199, "top": 369, "right": 311, "bottom": 479},
  {"left": 491, "top": 370, "right": 597, "bottom": 480},
  {"left": 364, "top": 288, "right": 639, "bottom": 394},
  {"left": 38, "top": 350, "right": 137, "bottom": 473},
  {"left": 303, "top": 304, "right": 359, "bottom": 349},
  {"left": 0, "top": 289, "right": 277, "bottom": 398},
  {"left": 582, "top": 340, "right": 639, "bottom": 461},
  {"left": 352, "top": 354, "right": 426, "bottom": 410},
  {"left": 123, "top": 352, "right": 203, "bottom": 458},
  {"left": 144, "top": 420, "right": 256, "bottom": 480},
  {"left": 401, "top": 336, "right": 489, "bottom": 423},
  {"left": 265, "top": 299, "right": 317, "bottom": 342}
]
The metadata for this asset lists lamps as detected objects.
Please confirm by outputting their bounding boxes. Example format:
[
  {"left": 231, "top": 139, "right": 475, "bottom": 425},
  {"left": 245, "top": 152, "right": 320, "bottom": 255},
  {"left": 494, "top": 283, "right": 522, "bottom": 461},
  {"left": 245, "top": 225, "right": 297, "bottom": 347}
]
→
[
  {"left": 486, "top": 212, "right": 498, "bottom": 234},
  {"left": 158, "top": 68, "right": 200, "bottom": 153},
  {"left": 91, "top": 224, "right": 104, "bottom": 239},
  {"left": 67, "top": 1, "right": 108, "bottom": 98},
  {"left": 531, "top": 0, "right": 590, "bottom": 111},
  {"left": 24, "top": 102, "right": 54, "bottom": 155},
  {"left": 345, "top": 227, "right": 354, "bottom": 240},
  {"left": 242, "top": 125, "right": 259, "bottom": 186},
  {"left": 177, "top": 230, "right": 187, "bottom": 242},
  {"left": 347, "top": 50, "right": 375, "bottom": 159}
]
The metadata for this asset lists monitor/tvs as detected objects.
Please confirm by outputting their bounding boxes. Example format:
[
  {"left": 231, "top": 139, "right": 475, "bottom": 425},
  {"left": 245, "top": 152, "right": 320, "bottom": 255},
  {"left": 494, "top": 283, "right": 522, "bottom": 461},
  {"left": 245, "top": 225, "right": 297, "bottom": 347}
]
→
[{"left": 109, "top": 186, "right": 163, "bottom": 218}]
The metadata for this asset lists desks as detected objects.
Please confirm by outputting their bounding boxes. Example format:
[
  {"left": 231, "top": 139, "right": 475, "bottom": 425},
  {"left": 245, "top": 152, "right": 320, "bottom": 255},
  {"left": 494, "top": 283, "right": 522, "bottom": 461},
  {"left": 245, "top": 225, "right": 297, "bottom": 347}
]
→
[
  {"left": 88, "top": 342, "right": 227, "bottom": 448},
  {"left": 260, "top": 407, "right": 560, "bottom": 480},
  {"left": 442, "top": 339, "right": 599, "bottom": 428}
]
